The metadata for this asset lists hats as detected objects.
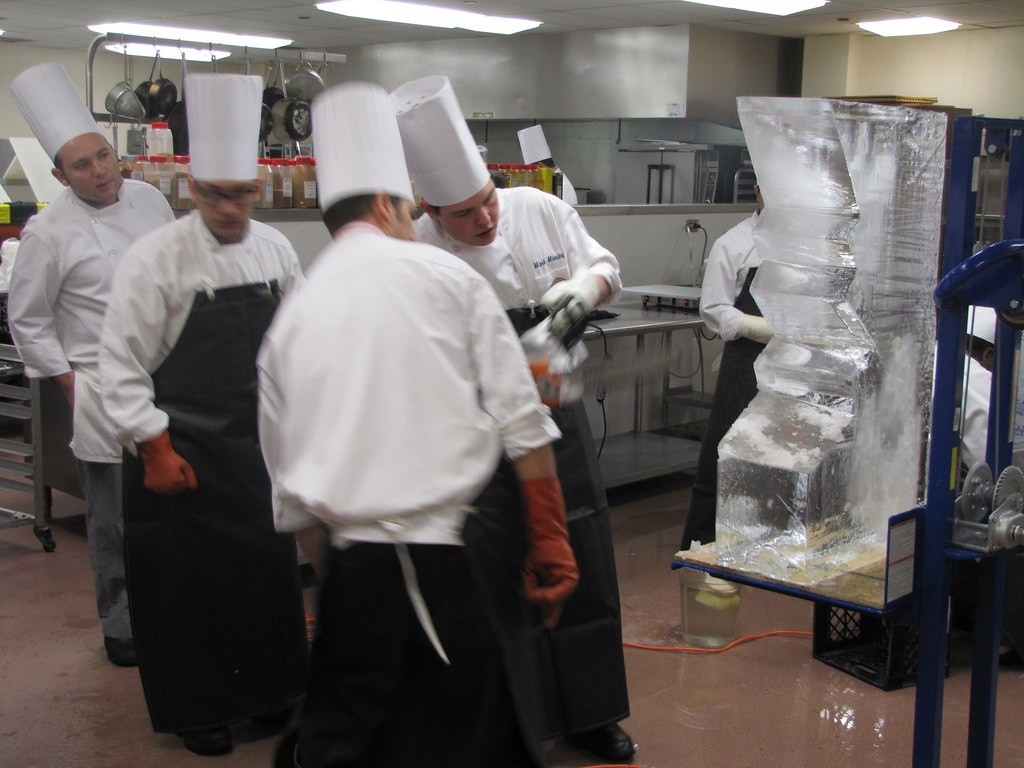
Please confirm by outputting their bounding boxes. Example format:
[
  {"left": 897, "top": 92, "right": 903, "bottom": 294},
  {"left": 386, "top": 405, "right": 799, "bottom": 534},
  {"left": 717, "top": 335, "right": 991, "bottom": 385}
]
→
[
  {"left": 967, "top": 303, "right": 997, "bottom": 346},
  {"left": 186, "top": 73, "right": 263, "bottom": 181},
  {"left": 312, "top": 82, "right": 416, "bottom": 218},
  {"left": 517, "top": 125, "right": 552, "bottom": 166},
  {"left": 392, "top": 73, "right": 491, "bottom": 208},
  {"left": 10, "top": 63, "right": 102, "bottom": 168}
]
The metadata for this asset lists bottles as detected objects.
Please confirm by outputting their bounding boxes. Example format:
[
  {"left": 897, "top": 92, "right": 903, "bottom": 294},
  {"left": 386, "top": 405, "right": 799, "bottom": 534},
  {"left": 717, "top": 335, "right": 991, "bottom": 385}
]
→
[
  {"left": 488, "top": 162, "right": 545, "bottom": 190},
  {"left": 119, "top": 154, "right": 319, "bottom": 210},
  {"left": 146, "top": 122, "right": 174, "bottom": 158}
]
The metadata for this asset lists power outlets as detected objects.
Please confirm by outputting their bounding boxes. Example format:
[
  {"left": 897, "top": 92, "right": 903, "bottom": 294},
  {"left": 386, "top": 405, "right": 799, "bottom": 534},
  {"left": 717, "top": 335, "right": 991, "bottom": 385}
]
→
[{"left": 686, "top": 219, "right": 699, "bottom": 232}]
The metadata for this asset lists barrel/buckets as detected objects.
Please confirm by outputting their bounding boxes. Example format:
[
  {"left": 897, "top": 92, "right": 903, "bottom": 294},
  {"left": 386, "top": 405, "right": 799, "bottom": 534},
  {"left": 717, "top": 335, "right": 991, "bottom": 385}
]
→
[{"left": 678, "top": 568, "right": 743, "bottom": 649}]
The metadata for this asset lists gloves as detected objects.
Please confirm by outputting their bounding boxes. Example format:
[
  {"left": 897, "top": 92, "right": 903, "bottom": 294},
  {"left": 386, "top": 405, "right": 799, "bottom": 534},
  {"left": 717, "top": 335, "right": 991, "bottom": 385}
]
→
[
  {"left": 542, "top": 266, "right": 599, "bottom": 347},
  {"left": 522, "top": 474, "right": 581, "bottom": 629},
  {"left": 137, "top": 430, "right": 198, "bottom": 496}
]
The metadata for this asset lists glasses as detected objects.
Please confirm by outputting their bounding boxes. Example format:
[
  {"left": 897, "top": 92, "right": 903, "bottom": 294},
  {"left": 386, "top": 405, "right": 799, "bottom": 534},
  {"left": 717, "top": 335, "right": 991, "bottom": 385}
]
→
[{"left": 196, "top": 181, "right": 257, "bottom": 205}]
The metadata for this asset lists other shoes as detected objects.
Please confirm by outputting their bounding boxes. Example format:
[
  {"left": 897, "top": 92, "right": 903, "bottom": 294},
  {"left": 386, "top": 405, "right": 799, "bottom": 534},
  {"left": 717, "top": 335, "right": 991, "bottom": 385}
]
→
[
  {"left": 566, "top": 722, "right": 635, "bottom": 762},
  {"left": 178, "top": 725, "right": 232, "bottom": 755},
  {"left": 104, "top": 636, "right": 137, "bottom": 666}
]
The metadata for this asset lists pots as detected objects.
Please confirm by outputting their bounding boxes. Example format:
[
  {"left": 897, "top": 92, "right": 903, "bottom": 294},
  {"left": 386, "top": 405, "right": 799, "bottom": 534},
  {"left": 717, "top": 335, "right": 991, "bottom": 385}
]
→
[{"left": 134, "top": 50, "right": 327, "bottom": 156}]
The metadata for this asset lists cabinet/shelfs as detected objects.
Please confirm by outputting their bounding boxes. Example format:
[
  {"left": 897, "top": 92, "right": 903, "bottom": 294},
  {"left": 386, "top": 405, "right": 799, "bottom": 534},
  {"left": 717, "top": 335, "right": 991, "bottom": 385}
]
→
[{"left": 583, "top": 303, "right": 705, "bottom": 489}]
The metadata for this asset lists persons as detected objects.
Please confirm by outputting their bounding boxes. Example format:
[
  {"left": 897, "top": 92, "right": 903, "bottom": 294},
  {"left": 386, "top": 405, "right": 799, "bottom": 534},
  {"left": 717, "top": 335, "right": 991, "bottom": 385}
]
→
[
  {"left": 518, "top": 124, "right": 577, "bottom": 204},
  {"left": 681, "top": 178, "right": 775, "bottom": 553},
  {"left": 255, "top": 85, "right": 580, "bottom": 768},
  {"left": 391, "top": 74, "right": 638, "bottom": 768},
  {"left": 7, "top": 61, "right": 177, "bottom": 665},
  {"left": 931, "top": 305, "right": 997, "bottom": 482},
  {"left": 97, "top": 72, "right": 307, "bottom": 757}
]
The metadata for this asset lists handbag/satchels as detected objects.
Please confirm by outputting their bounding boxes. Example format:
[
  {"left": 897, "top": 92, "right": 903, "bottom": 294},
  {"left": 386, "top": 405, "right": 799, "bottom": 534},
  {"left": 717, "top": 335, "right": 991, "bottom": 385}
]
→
[{"left": 518, "top": 315, "right": 588, "bottom": 409}]
